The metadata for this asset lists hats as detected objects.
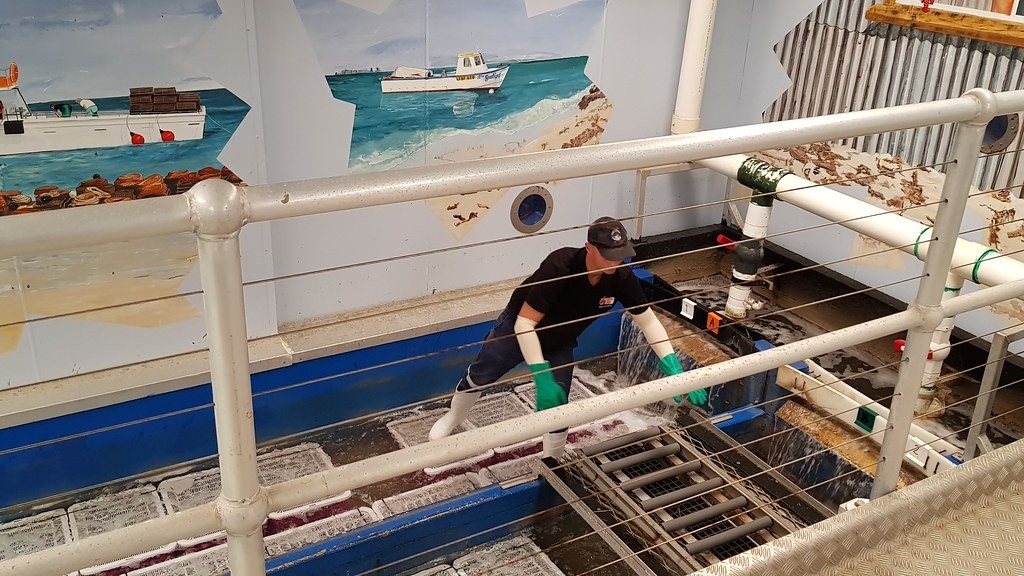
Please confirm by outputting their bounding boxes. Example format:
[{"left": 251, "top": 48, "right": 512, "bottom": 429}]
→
[{"left": 588, "top": 217, "right": 636, "bottom": 260}]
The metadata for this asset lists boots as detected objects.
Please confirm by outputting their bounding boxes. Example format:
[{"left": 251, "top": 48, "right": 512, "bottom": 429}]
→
[
  {"left": 543, "top": 426, "right": 569, "bottom": 462},
  {"left": 429, "top": 378, "right": 481, "bottom": 441}
]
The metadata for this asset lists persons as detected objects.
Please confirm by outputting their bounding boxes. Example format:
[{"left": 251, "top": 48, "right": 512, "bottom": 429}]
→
[{"left": 428, "top": 216, "right": 709, "bottom": 464}]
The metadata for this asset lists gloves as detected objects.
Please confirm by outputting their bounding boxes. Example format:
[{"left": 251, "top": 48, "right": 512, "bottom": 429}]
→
[
  {"left": 528, "top": 361, "right": 568, "bottom": 411},
  {"left": 657, "top": 353, "right": 708, "bottom": 405}
]
